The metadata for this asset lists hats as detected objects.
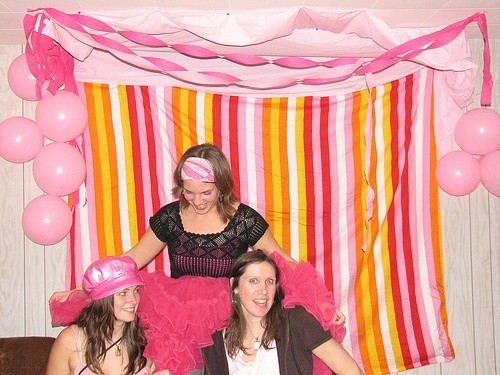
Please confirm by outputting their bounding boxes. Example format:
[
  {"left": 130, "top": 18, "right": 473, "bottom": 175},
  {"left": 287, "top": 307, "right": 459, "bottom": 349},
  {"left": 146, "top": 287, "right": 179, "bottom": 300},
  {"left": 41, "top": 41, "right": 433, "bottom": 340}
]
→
[{"left": 83, "top": 255, "right": 147, "bottom": 300}]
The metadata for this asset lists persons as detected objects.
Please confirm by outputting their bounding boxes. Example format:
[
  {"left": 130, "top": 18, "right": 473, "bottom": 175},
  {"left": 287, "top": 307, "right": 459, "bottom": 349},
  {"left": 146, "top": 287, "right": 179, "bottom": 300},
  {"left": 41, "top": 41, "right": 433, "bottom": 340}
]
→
[
  {"left": 49, "top": 144, "right": 346, "bottom": 375},
  {"left": 201, "top": 251, "right": 363, "bottom": 375},
  {"left": 45, "top": 255, "right": 171, "bottom": 375}
]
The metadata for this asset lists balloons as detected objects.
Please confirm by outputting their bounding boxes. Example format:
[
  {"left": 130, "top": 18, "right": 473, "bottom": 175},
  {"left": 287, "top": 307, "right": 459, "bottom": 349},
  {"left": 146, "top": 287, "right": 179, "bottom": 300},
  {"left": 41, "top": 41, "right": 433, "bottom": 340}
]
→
[
  {"left": 436, "top": 151, "right": 481, "bottom": 196},
  {"left": 8, "top": 53, "right": 54, "bottom": 102},
  {"left": 33, "top": 142, "right": 86, "bottom": 196},
  {"left": 22, "top": 196, "right": 72, "bottom": 246},
  {"left": 36, "top": 89, "right": 87, "bottom": 143},
  {"left": 0, "top": 116, "right": 44, "bottom": 164},
  {"left": 454, "top": 108, "right": 500, "bottom": 155},
  {"left": 480, "top": 151, "right": 500, "bottom": 197}
]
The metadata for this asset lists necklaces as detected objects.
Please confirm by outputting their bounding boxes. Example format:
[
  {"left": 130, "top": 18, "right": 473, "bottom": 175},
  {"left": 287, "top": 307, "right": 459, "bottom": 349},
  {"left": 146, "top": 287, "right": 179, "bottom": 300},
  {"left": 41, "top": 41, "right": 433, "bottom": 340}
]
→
[
  {"left": 110, "top": 338, "right": 122, "bottom": 356},
  {"left": 246, "top": 328, "right": 263, "bottom": 350}
]
